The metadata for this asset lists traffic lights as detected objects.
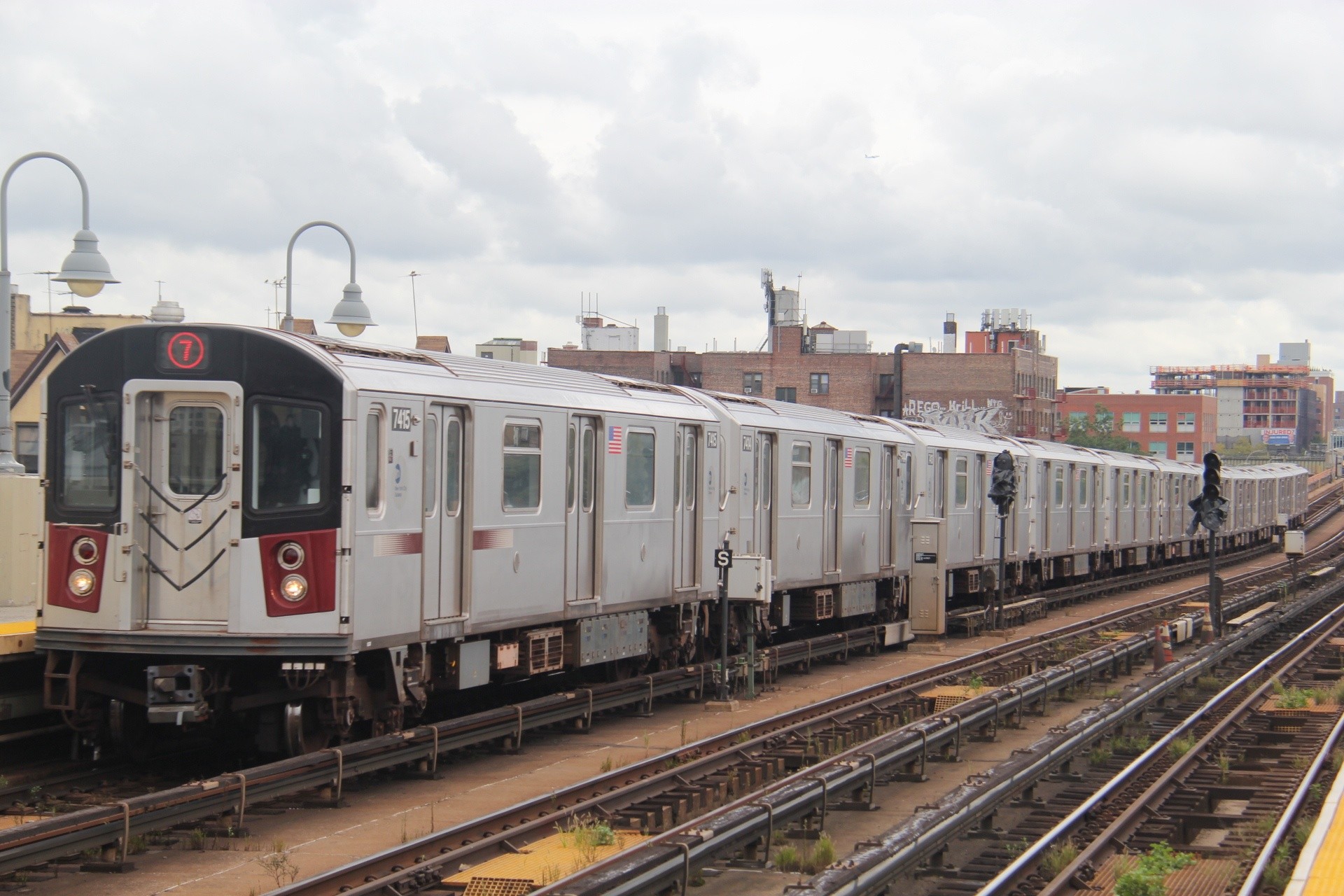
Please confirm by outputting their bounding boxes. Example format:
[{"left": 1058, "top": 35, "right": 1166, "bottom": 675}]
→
[{"left": 1203, "top": 451, "right": 1221, "bottom": 501}]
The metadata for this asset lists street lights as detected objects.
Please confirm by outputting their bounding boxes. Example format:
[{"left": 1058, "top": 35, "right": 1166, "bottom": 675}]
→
[
  {"left": 0, "top": 152, "right": 123, "bottom": 475},
  {"left": 408, "top": 270, "right": 420, "bottom": 338},
  {"left": 280, "top": 220, "right": 380, "bottom": 342},
  {"left": 31, "top": 271, "right": 61, "bottom": 338}
]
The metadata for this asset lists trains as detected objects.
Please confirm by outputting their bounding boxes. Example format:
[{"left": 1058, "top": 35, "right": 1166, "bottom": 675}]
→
[{"left": 23, "top": 320, "right": 1314, "bottom": 767}]
